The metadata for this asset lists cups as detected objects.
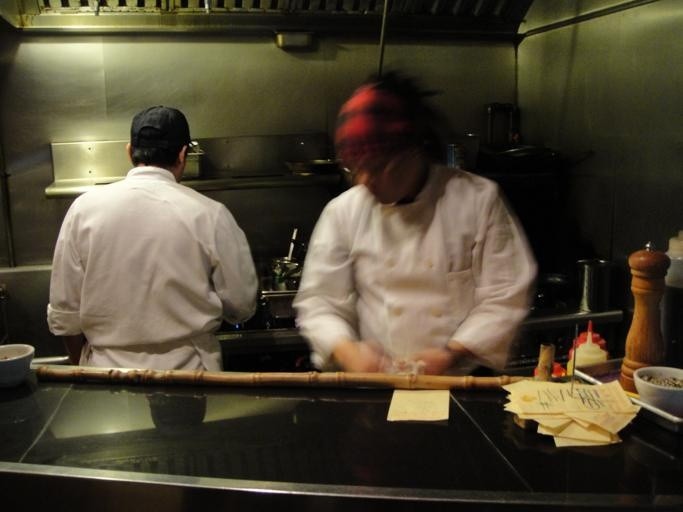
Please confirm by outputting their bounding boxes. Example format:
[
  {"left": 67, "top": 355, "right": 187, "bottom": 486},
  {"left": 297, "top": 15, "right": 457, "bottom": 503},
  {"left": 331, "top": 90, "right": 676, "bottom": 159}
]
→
[{"left": 271, "top": 257, "right": 296, "bottom": 292}]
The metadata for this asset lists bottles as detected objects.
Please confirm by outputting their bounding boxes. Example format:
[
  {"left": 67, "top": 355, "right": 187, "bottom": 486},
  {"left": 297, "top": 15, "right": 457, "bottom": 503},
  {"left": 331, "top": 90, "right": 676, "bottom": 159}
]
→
[
  {"left": 533, "top": 319, "right": 610, "bottom": 375},
  {"left": 661, "top": 229, "right": 683, "bottom": 365},
  {"left": 445, "top": 143, "right": 465, "bottom": 173}
]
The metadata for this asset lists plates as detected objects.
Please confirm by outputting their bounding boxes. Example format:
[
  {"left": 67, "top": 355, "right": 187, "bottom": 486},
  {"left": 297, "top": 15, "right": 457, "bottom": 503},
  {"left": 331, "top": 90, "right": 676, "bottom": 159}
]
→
[
  {"left": 32, "top": 354, "right": 71, "bottom": 366},
  {"left": 282, "top": 159, "right": 338, "bottom": 177}
]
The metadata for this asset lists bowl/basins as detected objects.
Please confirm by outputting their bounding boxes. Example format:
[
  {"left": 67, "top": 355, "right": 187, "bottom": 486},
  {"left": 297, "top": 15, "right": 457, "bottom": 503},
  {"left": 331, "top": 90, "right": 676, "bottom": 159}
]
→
[
  {"left": 0, "top": 344, "right": 33, "bottom": 390},
  {"left": 632, "top": 366, "right": 682, "bottom": 415},
  {"left": 192, "top": 131, "right": 311, "bottom": 181}
]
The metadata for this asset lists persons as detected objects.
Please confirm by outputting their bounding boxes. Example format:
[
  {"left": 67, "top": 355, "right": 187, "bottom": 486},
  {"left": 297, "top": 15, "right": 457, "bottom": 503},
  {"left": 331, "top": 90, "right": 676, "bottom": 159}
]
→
[
  {"left": 291, "top": 64, "right": 539, "bottom": 376},
  {"left": 47, "top": 106, "right": 259, "bottom": 373}
]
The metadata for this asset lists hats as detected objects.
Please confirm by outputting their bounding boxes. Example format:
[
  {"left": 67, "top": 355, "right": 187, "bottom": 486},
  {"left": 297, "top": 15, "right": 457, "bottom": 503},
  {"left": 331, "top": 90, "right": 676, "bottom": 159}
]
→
[{"left": 130, "top": 105, "right": 197, "bottom": 154}]
